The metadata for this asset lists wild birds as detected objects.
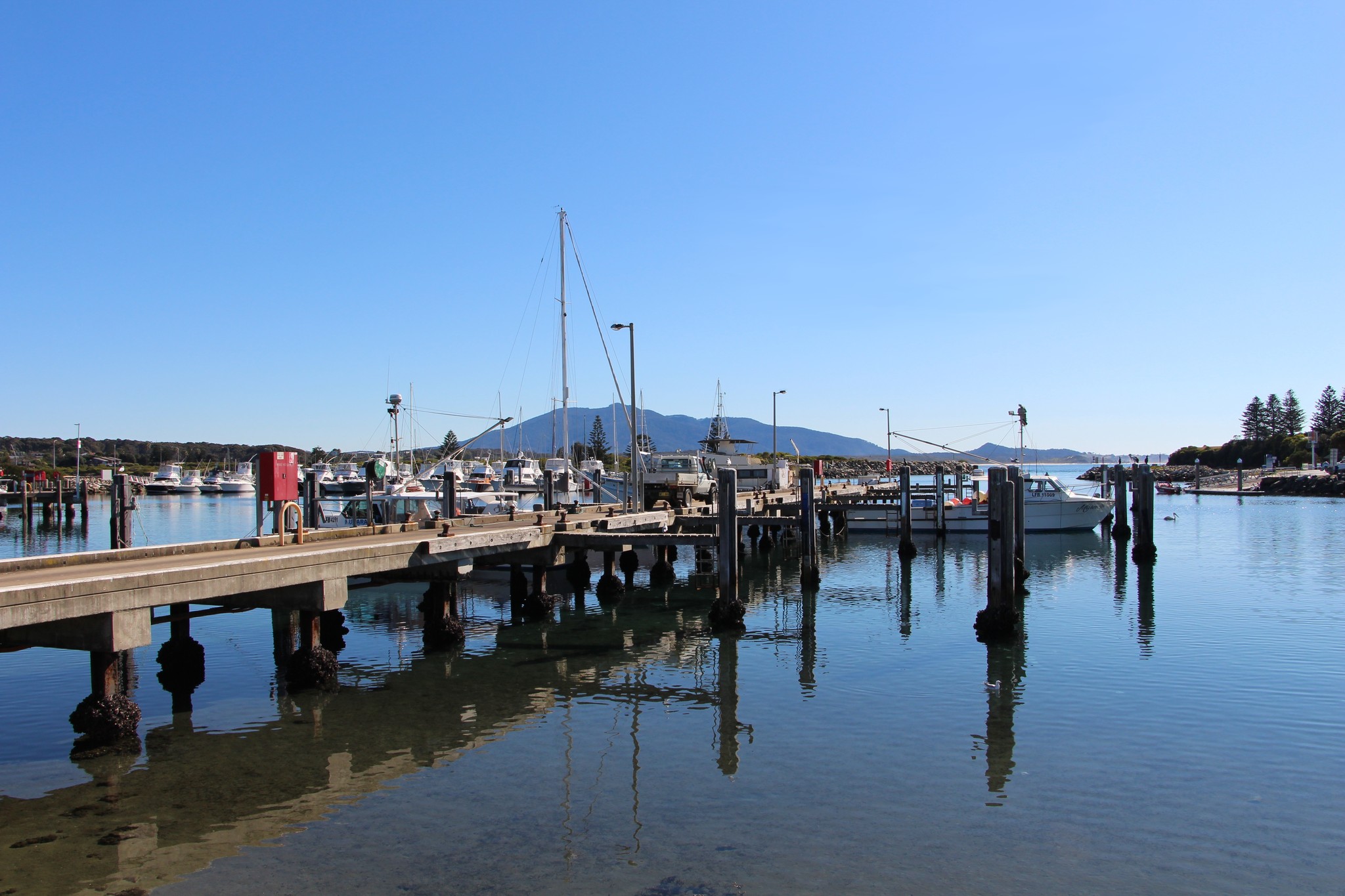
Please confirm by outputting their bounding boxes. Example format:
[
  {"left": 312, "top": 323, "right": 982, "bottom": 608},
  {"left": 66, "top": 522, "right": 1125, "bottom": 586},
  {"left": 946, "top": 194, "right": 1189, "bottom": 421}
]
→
[
  {"left": 662, "top": 522, "right": 668, "bottom": 534},
  {"left": 725, "top": 458, "right": 732, "bottom": 468},
  {"left": 1163, "top": 512, "right": 1180, "bottom": 521},
  {"left": 1144, "top": 455, "right": 1149, "bottom": 464},
  {"left": 983, "top": 680, "right": 1002, "bottom": 690},
  {"left": 1128, "top": 453, "right": 1139, "bottom": 464},
  {"left": 1118, "top": 456, "right": 1121, "bottom": 464},
  {"left": 1008, "top": 457, "right": 1020, "bottom": 466},
  {"left": 118, "top": 466, "right": 125, "bottom": 474},
  {"left": 902, "top": 458, "right": 908, "bottom": 466},
  {"left": 867, "top": 478, "right": 881, "bottom": 486}
]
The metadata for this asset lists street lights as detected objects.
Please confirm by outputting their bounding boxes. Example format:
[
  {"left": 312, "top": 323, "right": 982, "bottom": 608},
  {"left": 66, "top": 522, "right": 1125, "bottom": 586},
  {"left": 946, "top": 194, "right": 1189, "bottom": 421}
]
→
[
  {"left": 74, "top": 423, "right": 82, "bottom": 502},
  {"left": 605, "top": 319, "right": 642, "bottom": 516},
  {"left": 879, "top": 408, "right": 891, "bottom": 461},
  {"left": 765, "top": 389, "right": 789, "bottom": 494},
  {"left": 51, "top": 438, "right": 58, "bottom": 470}
]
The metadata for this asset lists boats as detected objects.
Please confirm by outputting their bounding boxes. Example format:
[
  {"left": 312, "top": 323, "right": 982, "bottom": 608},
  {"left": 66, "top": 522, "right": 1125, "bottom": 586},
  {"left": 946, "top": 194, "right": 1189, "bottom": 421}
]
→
[
  {"left": 1155, "top": 480, "right": 1182, "bottom": 494},
  {"left": 844, "top": 474, "right": 1116, "bottom": 532},
  {"left": 142, "top": 446, "right": 645, "bottom": 529}
]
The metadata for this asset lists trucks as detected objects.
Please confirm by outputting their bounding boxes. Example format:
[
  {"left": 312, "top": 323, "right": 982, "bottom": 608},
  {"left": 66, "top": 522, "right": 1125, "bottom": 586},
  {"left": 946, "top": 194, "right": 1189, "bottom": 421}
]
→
[{"left": 627, "top": 452, "right": 718, "bottom": 509}]
